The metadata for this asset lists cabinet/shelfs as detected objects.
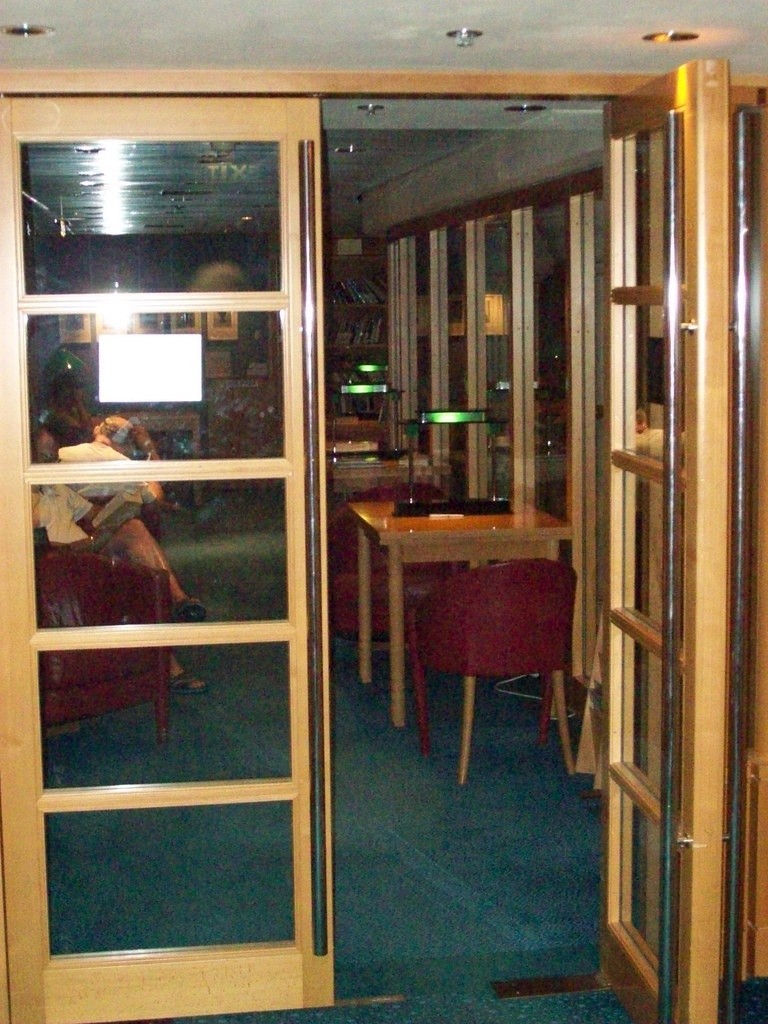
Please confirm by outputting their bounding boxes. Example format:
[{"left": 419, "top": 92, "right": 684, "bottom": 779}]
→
[{"left": 265, "top": 251, "right": 388, "bottom": 380}]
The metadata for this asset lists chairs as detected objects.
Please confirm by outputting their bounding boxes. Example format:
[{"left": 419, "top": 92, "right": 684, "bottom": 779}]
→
[
  {"left": 36, "top": 550, "right": 170, "bottom": 746},
  {"left": 415, "top": 558, "right": 578, "bottom": 785},
  {"left": 328, "top": 484, "right": 456, "bottom": 636}
]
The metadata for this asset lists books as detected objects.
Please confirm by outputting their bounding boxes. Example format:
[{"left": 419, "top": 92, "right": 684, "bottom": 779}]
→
[
  {"left": 90, "top": 486, "right": 144, "bottom": 528},
  {"left": 331, "top": 270, "right": 388, "bottom": 304},
  {"left": 398, "top": 453, "right": 430, "bottom": 467},
  {"left": 336, "top": 392, "right": 375, "bottom": 414},
  {"left": 333, "top": 312, "right": 385, "bottom": 345},
  {"left": 327, "top": 355, "right": 386, "bottom": 384},
  {"left": 335, "top": 456, "right": 383, "bottom": 470}
]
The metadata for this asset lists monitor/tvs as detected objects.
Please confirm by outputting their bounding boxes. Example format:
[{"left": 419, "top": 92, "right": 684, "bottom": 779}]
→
[{"left": 96, "top": 332, "right": 205, "bottom": 407}]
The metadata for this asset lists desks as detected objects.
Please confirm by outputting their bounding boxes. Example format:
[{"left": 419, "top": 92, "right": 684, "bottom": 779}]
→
[
  {"left": 346, "top": 501, "right": 573, "bottom": 728},
  {"left": 104, "top": 409, "right": 203, "bottom": 507}
]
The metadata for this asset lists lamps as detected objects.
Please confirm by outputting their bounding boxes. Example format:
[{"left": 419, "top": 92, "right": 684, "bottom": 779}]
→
[
  {"left": 403, "top": 408, "right": 509, "bottom": 501},
  {"left": 330, "top": 381, "right": 401, "bottom": 465}
]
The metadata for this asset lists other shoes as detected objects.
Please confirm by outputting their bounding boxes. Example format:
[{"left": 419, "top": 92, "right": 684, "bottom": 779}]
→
[
  {"left": 169, "top": 672, "right": 209, "bottom": 694},
  {"left": 173, "top": 598, "right": 207, "bottom": 622}
]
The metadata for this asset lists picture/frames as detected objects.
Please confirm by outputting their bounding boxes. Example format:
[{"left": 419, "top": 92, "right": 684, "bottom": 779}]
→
[{"left": 54, "top": 310, "right": 245, "bottom": 352}]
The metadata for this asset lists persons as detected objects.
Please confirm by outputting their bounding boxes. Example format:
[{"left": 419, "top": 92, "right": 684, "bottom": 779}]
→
[
  {"left": 27, "top": 426, "right": 211, "bottom": 693},
  {"left": 58, "top": 415, "right": 167, "bottom": 509},
  {"left": 634, "top": 408, "right": 666, "bottom": 460}
]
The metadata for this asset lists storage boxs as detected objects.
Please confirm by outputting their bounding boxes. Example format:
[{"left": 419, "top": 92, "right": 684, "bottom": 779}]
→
[{"left": 336, "top": 238, "right": 363, "bottom": 254}]
